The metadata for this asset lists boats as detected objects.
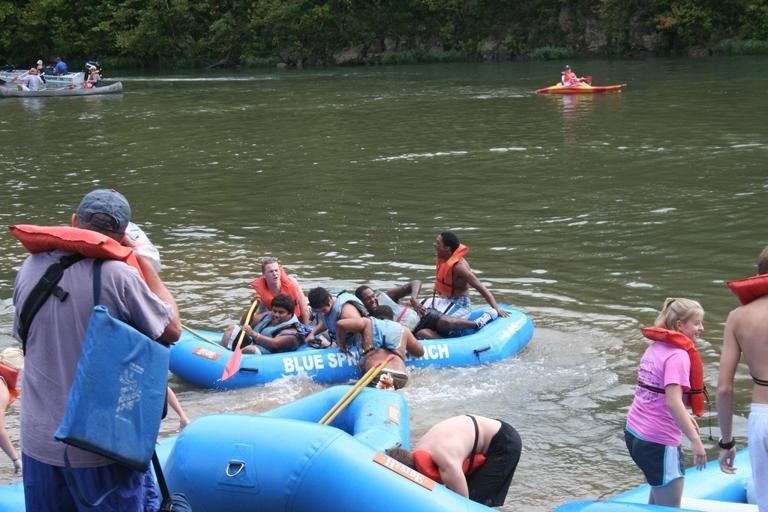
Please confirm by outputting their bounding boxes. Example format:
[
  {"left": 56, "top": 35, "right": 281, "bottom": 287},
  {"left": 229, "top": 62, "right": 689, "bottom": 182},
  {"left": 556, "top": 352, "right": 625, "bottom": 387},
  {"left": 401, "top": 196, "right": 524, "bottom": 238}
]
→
[
  {"left": 0, "top": 386, "right": 413, "bottom": 511},
  {"left": 163, "top": 413, "right": 504, "bottom": 511},
  {"left": 552, "top": 442, "right": 758, "bottom": 511},
  {"left": 1, "top": 68, "right": 86, "bottom": 87},
  {"left": 535, "top": 81, "right": 628, "bottom": 96},
  {"left": 1, "top": 82, "right": 124, "bottom": 97},
  {"left": 169, "top": 297, "right": 534, "bottom": 390}
]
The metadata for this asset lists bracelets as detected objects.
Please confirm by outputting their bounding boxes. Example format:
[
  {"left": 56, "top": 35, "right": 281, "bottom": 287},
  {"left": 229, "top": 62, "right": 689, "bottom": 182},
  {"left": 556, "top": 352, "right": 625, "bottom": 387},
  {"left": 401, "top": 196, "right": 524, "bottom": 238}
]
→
[{"left": 718, "top": 437, "right": 736, "bottom": 449}]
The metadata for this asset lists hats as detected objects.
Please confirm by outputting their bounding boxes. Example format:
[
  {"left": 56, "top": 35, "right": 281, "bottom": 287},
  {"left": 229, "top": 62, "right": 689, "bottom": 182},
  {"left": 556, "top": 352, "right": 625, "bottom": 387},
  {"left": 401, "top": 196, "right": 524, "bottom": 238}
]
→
[{"left": 77, "top": 189, "right": 130, "bottom": 234}]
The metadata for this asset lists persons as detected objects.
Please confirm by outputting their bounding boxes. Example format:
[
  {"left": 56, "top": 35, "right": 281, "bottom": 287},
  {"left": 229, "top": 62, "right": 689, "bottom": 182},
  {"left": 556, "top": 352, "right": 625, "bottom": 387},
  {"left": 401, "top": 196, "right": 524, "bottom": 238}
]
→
[
  {"left": 423, "top": 234, "right": 512, "bottom": 318},
  {"left": 384, "top": 413, "right": 522, "bottom": 508},
  {"left": 624, "top": 297, "right": 707, "bottom": 508},
  {"left": 17, "top": 57, "right": 103, "bottom": 92},
  {"left": 715, "top": 248, "right": 768, "bottom": 511},
  {"left": 222, "top": 293, "right": 305, "bottom": 354},
  {"left": 12, "top": 190, "right": 182, "bottom": 512},
  {"left": 355, "top": 280, "right": 498, "bottom": 340},
  {"left": 251, "top": 259, "right": 309, "bottom": 323},
  {"left": 305, "top": 287, "right": 369, "bottom": 348},
  {"left": 333, "top": 304, "right": 423, "bottom": 387},
  {"left": 562, "top": 64, "right": 589, "bottom": 86}
]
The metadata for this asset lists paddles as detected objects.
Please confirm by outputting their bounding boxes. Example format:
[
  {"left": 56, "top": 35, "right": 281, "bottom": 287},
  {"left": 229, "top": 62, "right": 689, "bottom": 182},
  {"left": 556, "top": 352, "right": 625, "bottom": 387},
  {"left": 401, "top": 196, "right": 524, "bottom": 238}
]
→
[
  {"left": 576, "top": 76, "right": 591, "bottom": 83},
  {"left": 10, "top": 69, "right": 30, "bottom": 83},
  {"left": 221, "top": 300, "right": 258, "bottom": 382}
]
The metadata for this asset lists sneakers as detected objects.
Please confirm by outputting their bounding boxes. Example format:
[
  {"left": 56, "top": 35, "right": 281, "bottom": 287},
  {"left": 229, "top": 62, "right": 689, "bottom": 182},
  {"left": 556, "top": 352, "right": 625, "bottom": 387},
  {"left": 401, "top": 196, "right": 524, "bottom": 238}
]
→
[{"left": 473, "top": 310, "right": 498, "bottom": 328}]
